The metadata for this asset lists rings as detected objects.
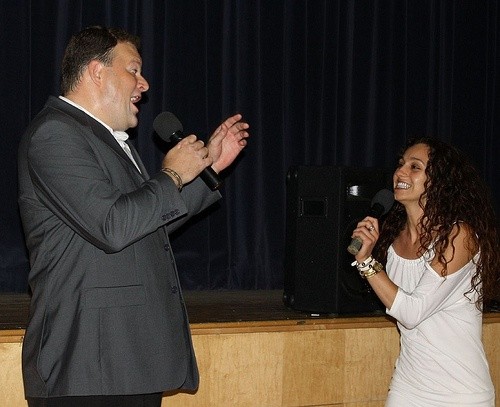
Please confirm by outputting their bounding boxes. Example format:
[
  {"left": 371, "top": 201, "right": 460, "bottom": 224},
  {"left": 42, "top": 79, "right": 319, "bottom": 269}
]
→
[{"left": 368, "top": 225, "right": 375, "bottom": 231}]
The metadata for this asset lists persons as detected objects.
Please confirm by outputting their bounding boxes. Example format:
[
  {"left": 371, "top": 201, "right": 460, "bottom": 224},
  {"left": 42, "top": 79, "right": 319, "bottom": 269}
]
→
[
  {"left": 15, "top": 26, "right": 251, "bottom": 407},
  {"left": 351, "top": 137, "right": 500, "bottom": 407}
]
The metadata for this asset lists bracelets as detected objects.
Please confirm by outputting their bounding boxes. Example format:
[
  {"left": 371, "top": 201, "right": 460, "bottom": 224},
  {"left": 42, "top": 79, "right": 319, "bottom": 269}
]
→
[
  {"left": 161, "top": 166, "right": 184, "bottom": 191},
  {"left": 351, "top": 258, "right": 386, "bottom": 278}
]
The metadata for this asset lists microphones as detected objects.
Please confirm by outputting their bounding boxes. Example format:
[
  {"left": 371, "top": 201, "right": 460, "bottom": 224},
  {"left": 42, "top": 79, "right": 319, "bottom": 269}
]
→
[
  {"left": 346, "top": 190, "right": 395, "bottom": 255},
  {"left": 153, "top": 112, "right": 223, "bottom": 190}
]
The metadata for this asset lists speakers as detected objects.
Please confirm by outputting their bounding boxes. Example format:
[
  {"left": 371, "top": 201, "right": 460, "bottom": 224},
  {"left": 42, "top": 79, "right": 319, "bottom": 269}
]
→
[{"left": 284, "top": 165, "right": 396, "bottom": 319}]
